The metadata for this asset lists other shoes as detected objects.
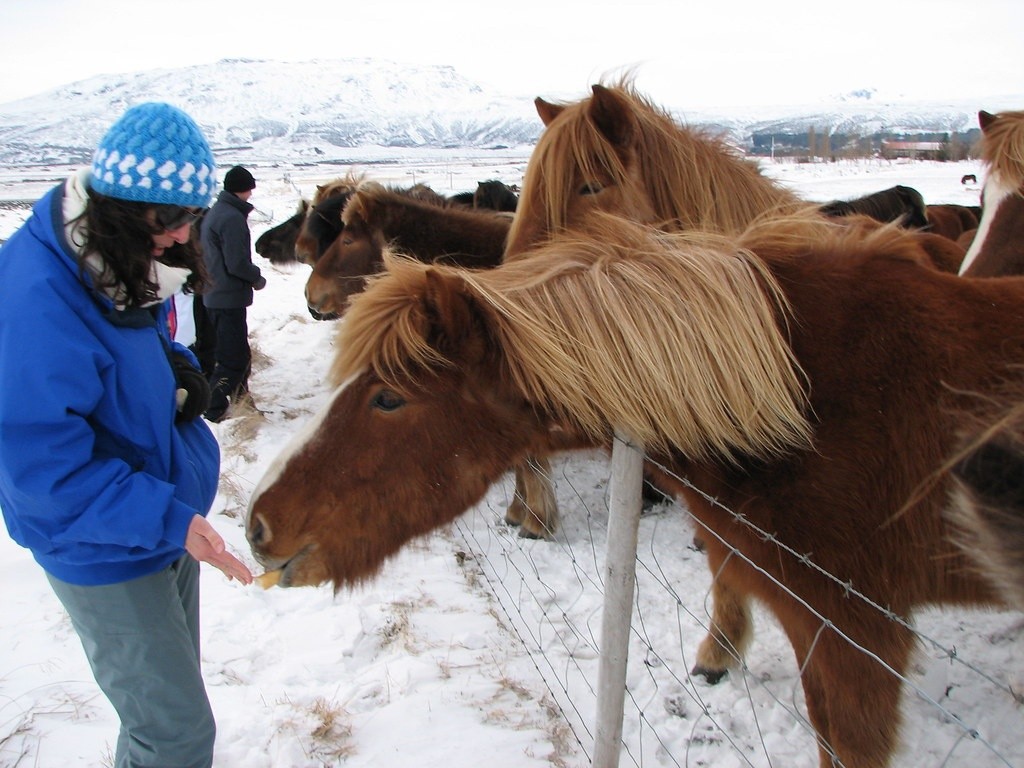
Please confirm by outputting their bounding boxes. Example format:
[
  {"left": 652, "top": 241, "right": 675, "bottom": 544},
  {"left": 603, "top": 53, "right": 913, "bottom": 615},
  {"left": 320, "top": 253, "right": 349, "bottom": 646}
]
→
[{"left": 206, "top": 391, "right": 228, "bottom": 421}]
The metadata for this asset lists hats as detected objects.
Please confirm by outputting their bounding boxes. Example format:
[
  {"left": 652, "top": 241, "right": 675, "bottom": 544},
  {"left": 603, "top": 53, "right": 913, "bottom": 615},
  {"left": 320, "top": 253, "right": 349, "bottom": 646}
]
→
[
  {"left": 89, "top": 101, "right": 216, "bottom": 209},
  {"left": 224, "top": 166, "right": 256, "bottom": 192}
]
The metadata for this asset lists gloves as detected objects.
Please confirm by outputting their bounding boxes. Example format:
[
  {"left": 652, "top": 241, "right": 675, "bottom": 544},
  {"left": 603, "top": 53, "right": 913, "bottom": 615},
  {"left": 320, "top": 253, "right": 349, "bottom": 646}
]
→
[{"left": 168, "top": 351, "right": 208, "bottom": 425}]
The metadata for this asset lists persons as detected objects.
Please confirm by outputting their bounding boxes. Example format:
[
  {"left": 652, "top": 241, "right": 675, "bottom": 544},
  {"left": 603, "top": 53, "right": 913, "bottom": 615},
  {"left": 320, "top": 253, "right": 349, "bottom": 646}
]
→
[
  {"left": 0, "top": 102, "right": 253, "bottom": 768},
  {"left": 183, "top": 167, "right": 268, "bottom": 423}
]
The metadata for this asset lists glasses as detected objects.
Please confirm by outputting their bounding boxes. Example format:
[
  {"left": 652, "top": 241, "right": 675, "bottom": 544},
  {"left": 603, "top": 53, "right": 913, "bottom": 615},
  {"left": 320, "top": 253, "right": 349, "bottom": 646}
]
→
[{"left": 154, "top": 204, "right": 201, "bottom": 232}]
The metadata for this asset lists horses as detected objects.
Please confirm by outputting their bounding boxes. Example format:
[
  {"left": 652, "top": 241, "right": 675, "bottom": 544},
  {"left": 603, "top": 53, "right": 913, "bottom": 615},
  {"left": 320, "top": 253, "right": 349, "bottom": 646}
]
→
[{"left": 241, "top": 80, "right": 1024, "bottom": 768}]
